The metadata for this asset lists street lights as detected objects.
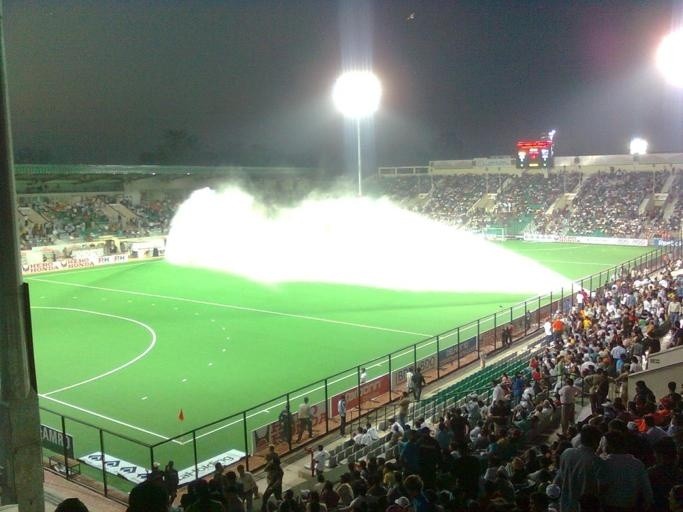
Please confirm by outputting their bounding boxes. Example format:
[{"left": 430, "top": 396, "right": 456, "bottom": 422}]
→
[{"left": 327, "top": 67, "right": 385, "bottom": 194}]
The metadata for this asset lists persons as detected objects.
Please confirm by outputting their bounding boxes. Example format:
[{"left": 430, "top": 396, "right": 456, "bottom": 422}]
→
[
  {"left": 127, "top": 250, "right": 683, "bottom": 512},
  {"left": 19, "top": 172, "right": 182, "bottom": 263},
  {"left": 379, "top": 166, "right": 682, "bottom": 242},
  {"left": 55, "top": 498, "right": 89, "bottom": 511}
]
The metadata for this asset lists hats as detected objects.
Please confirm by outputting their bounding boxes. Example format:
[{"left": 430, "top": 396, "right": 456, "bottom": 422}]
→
[
  {"left": 395, "top": 497, "right": 409, "bottom": 506},
  {"left": 546, "top": 484, "right": 561, "bottom": 499}
]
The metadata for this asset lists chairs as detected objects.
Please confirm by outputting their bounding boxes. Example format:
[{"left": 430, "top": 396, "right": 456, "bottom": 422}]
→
[{"left": 328, "top": 338, "right": 547, "bottom": 466}]
